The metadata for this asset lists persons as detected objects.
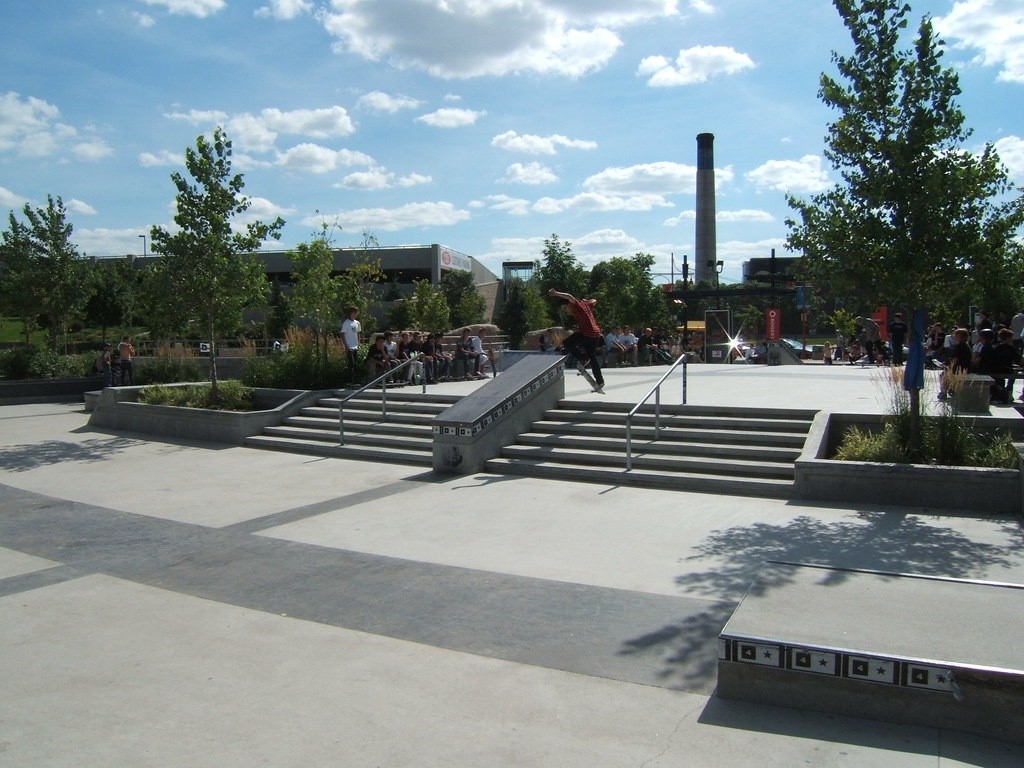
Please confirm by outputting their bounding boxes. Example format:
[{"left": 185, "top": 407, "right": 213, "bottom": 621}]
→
[
  {"left": 117, "top": 336, "right": 136, "bottom": 386},
  {"left": 539, "top": 324, "right": 675, "bottom": 365},
  {"left": 457, "top": 328, "right": 482, "bottom": 378},
  {"left": 745, "top": 341, "right": 767, "bottom": 362},
  {"left": 471, "top": 328, "right": 490, "bottom": 378},
  {"left": 823, "top": 309, "right": 1024, "bottom": 406},
  {"left": 339, "top": 306, "right": 362, "bottom": 389},
  {"left": 102, "top": 346, "right": 122, "bottom": 388},
  {"left": 364, "top": 332, "right": 402, "bottom": 387},
  {"left": 549, "top": 288, "right": 604, "bottom": 387},
  {"left": 423, "top": 334, "right": 449, "bottom": 384},
  {"left": 399, "top": 332, "right": 438, "bottom": 384}
]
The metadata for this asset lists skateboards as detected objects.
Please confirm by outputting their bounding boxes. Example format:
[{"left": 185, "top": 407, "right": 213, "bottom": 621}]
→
[
  {"left": 576, "top": 360, "right": 605, "bottom": 395},
  {"left": 377, "top": 380, "right": 410, "bottom": 388},
  {"left": 465, "top": 375, "right": 486, "bottom": 380},
  {"left": 410, "top": 352, "right": 421, "bottom": 385},
  {"left": 488, "top": 347, "right": 496, "bottom": 378}
]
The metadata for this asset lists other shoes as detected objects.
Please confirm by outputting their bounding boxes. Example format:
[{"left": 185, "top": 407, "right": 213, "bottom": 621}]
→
[
  {"left": 866, "top": 360, "right": 874, "bottom": 364},
  {"left": 388, "top": 378, "right": 394, "bottom": 384},
  {"left": 352, "top": 383, "right": 360, "bottom": 389},
  {"left": 595, "top": 381, "right": 604, "bottom": 388},
  {"left": 426, "top": 376, "right": 438, "bottom": 384},
  {"left": 475, "top": 371, "right": 483, "bottom": 376},
  {"left": 616, "top": 361, "right": 626, "bottom": 365},
  {"left": 581, "top": 357, "right": 591, "bottom": 366},
  {"left": 937, "top": 393, "right": 947, "bottom": 400},
  {"left": 344, "top": 383, "right": 352, "bottom": 389},
  {"left": 466, "top": 372, "right": 473, "bottom": 380},
  {"left": 625, "top": 361, "right": 631, "bottom": 364}
]
